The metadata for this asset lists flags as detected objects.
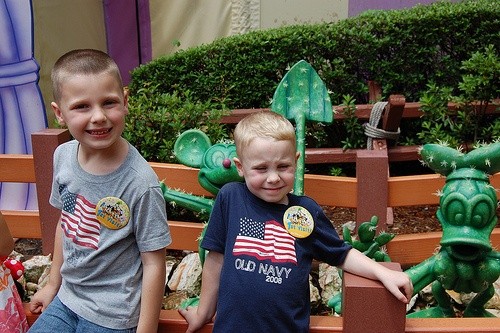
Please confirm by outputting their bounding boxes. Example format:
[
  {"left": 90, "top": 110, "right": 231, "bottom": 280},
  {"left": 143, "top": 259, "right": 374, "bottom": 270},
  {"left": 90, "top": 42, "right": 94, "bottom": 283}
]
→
[
  {"left": 231, "top": 215, "right": 298, "bottom": 266},
  {"left": 56, "top": 183, "right": 105, "bottom": 250}
]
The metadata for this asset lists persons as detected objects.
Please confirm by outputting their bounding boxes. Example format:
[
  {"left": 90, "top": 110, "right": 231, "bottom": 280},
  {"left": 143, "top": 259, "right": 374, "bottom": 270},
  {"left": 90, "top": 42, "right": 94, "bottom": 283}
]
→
[
  {"left": 0, "top": 211, "right": 31, "bottom": 333},
  {"left": 177, "top": 110, "right": 415, "bottom": 332},
  {"left": 21, "top": 47, "right": 173, "bottom": 333}
]
